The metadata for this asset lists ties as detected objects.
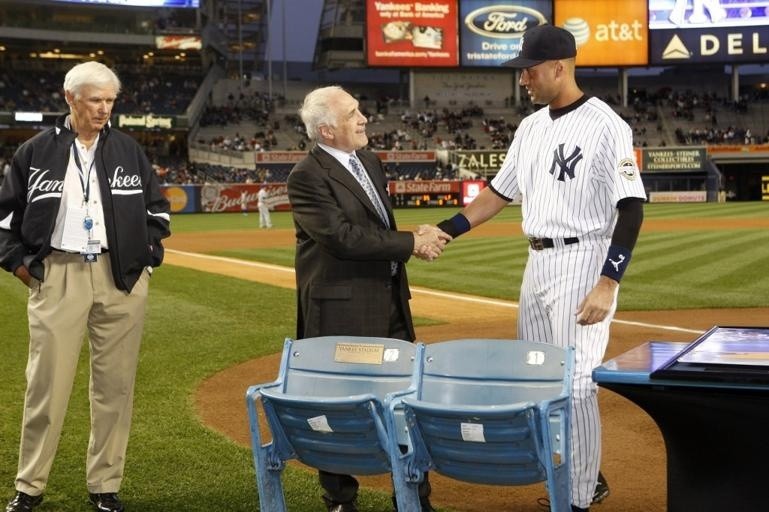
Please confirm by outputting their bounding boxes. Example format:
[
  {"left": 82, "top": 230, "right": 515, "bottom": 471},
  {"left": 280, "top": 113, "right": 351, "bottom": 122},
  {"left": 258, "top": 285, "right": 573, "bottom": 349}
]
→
[{"left": 349, "top": 154, "right": 398, "bottom": 276}]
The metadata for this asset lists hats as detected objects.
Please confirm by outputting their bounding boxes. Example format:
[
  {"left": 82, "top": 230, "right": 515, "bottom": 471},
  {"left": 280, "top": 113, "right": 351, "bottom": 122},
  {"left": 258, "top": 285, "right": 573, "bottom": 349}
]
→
[{"left": 501, "top": 25, "right": 576, "bottom": 68}]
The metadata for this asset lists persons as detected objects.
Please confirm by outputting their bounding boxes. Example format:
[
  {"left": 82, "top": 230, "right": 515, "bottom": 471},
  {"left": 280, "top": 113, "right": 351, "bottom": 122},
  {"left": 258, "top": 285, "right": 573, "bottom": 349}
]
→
[
  {"left": 419, "top": 22, "right": 649, "bottom": 512},
  {"left": 0, "top": 60, "right": 173, "bottom": 511},
  {"left": 2, "top": 64, "right": 769, "bottom": 190},
  {"left": 288, "top": 84, "right": 455, "bottom": 511},
  {"left": 239, "top": 191, "right": 252, "bottom": 216},
  {"left": 257, "top": 184, "right": 274, "bottom": 230}
]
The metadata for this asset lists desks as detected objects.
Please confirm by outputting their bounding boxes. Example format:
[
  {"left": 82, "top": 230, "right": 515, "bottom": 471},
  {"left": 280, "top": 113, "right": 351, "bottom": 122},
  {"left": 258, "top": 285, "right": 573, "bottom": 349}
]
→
[{"left": 592, "top": 341, "right": 768, "bottom": 512}]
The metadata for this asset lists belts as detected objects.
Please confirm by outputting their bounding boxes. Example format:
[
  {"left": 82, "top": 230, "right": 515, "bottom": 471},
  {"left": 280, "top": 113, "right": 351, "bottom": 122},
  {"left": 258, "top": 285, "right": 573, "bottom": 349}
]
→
[{"left": 530, "top": 238, "right": 579, "bottom": 250}]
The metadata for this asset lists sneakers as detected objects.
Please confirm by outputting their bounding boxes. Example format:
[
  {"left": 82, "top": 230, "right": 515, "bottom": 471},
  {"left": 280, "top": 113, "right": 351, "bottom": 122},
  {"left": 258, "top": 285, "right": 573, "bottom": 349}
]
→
[
  {"left": 6, "top": 491, "right": 43, "bottom": 512},
  {"left": 89, "top": 493, "right": 124, "bottom": 512},
  {"left": 592, "top": 470, "right": 609, "bottom": 502}
]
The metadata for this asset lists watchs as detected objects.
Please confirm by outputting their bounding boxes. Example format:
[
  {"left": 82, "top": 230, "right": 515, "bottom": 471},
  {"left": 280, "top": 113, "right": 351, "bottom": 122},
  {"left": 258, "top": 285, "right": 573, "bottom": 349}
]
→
[{"left": 146, "top": 265, "right": 154, "bottom": 275}]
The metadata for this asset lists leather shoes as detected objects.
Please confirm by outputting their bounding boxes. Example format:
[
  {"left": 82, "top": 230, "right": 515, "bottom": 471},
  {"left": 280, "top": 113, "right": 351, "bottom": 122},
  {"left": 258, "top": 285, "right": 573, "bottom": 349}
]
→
[{"left": 330, "top": 502, "right": 360, "bottom": 512}]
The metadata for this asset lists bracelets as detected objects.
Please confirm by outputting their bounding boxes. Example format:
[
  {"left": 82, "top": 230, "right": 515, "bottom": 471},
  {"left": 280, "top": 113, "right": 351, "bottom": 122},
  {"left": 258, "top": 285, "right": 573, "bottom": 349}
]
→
[
  {"left": 435, "top": 219, "right": 459, "bottom": 244},
  {"left": 598, "top": 242, "right": 632, "bottom": 285},
  {"left": 450, "top": 211, "right": 471, "bottom": 236}
]
